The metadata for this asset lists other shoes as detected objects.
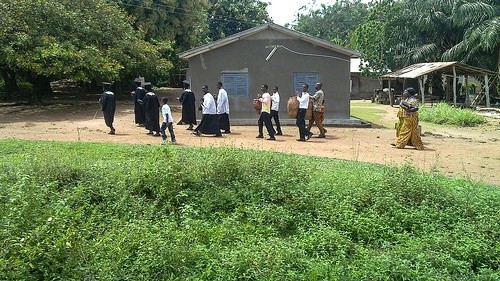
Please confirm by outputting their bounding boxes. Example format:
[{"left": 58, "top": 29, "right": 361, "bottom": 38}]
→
[
  {"left": 276, "top": 132, "right": 282, "bottom": 135},
  {"left": 306, "top": 133, "right": 313, "bottom": 140},
  {"left": 324, "top": 129, "right": 327, "bottom": 133},
  {"left": 187, "top": 127, "right": 194, "bottom": 130},
  {"left": 223, "top": 131, "right": 231, "bottom": 134},
  {"left": 148, "top": 132, "right": 153, "bottom": 134},
  {"left": 137, "top": 124, "right": 144, "bottom": 127},
  {"left": 296, "top": 138, "right": 305, "bottom": 141},
  {"left": 267, "top": 137, "right": 274, "bottom": 140},
  {"left": 163, "top": 138, "right": 168, "bottom": 142},
  {"left": 154, "top": 134, "right": 160, "bottom": 136},
  {"left": 316, "top": 134, "right": 325, "bottom": 138},
  {"left": 169, "top": 141, "right": 176, "bottom": 144},
  {"left": 108, "top": 128, "right": 115, "bottom": 134},
  {"left": 193, "top": 132, "right": 200, "bottom": 135},
  {"left": 256, "top": 134, "right": 263, "bottom": 138}
]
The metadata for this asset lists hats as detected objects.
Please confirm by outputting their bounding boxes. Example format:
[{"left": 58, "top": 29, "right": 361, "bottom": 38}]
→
[
  {"left": 143, "top": 82, "right": 151, "bottom": 91},
  {"left": 102, "top": 82, "right": 111, "bottom": 91},
  {"left": 183, "top": 80, "right": 190, "bottom": 87},
  {"left": 407, "top": 88, "right": 416, "bottom": 96},
  {"left": 134, "top": 78, "right": 141, "bottom": 85}
]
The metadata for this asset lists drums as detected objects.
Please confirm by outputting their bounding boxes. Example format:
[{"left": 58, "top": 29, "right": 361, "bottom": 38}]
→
[
  {"left": 286, "top": 96, "right": 299, "bottom": 120},
  {"left": 305, "top": 94, "right": 314, "bottom": 120},
  {"left": 253, "top": 95, "right": 262, "bottom": 116}
]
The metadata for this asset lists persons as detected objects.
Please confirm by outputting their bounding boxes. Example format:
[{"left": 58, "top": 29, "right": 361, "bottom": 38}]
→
[
  {"left": 255, "top": 84, "right": 275, "bottom": 140},
  {"left": 213, "top": 82, "right": 231, "bottom": 133},
  {"left": 390, "top": 76, "right": 476, "bottom": 150},
  {"left": 267, "top": 86, "right": 281, "bottom": 135},
  {"left": 99, "top": 78, "right": 196, "bottom": 145},
  {"left": 295, "top": 83, "right": 327, "bottom": 141},
  {"left": 192, "top": 84, "right": 222, "bottom": 137}
]
